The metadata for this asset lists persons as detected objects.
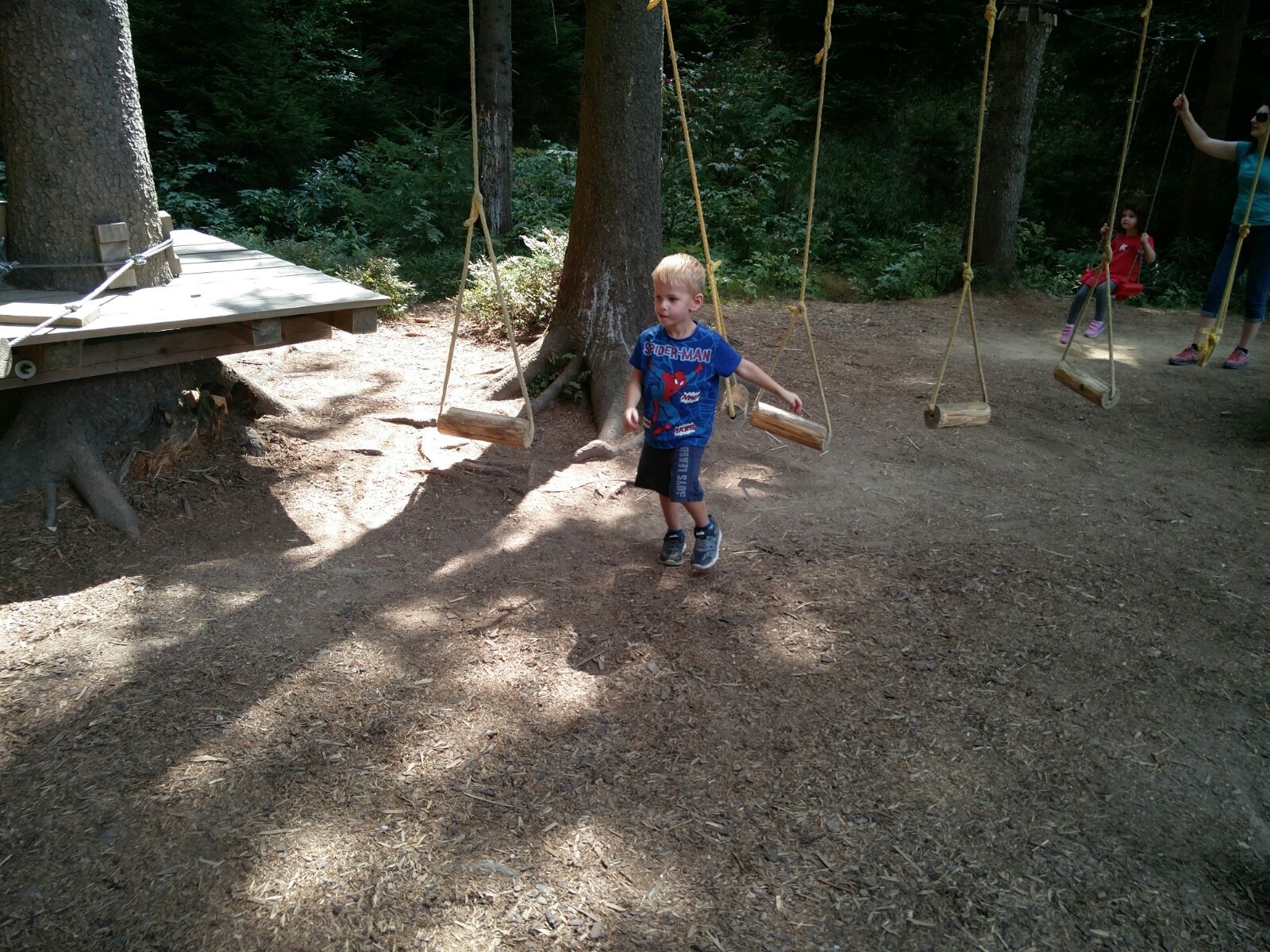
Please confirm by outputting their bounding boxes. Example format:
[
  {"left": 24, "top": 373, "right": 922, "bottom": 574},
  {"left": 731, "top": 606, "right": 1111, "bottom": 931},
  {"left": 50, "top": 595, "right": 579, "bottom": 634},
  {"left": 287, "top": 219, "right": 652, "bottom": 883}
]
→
[
  {"left": 1061, "top": 196, "right": 1156, "bottom": 346},
  {"left": 1167, "top": 91, "right": 1270, "bottom": 368},
  {"left": 622, "top": 253, "right": 805, "bottom": 571}
]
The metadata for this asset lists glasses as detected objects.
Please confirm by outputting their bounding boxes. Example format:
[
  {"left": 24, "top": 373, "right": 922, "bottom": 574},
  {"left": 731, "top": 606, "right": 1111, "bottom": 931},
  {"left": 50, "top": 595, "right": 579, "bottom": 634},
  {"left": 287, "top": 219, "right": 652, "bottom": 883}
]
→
[{"left": 1255, "top": 112, "right": 1270, "bottom": 122}]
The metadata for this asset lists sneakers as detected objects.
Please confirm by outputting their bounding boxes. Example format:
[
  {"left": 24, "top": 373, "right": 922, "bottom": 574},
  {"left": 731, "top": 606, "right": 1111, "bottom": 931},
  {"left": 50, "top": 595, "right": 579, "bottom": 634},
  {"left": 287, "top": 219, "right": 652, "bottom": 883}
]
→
[
  {"left": 1224, "top": 349, "right": 1249, "bottom": 369},
  {"left": 690, "top": 514, "right": 722, "bottom": 570},
  {"left": 1169, "top": 343, "right": 1204, "bottom": 366},
  {"left": 658, "top": 529, "right": 686, "bottom": 566},
  {"left": 1084, "top": 319, "right": 1105, "bottom": 337},
  {"left": 1060, "top": 323, "right": 1075, "bottom": 344}
]
